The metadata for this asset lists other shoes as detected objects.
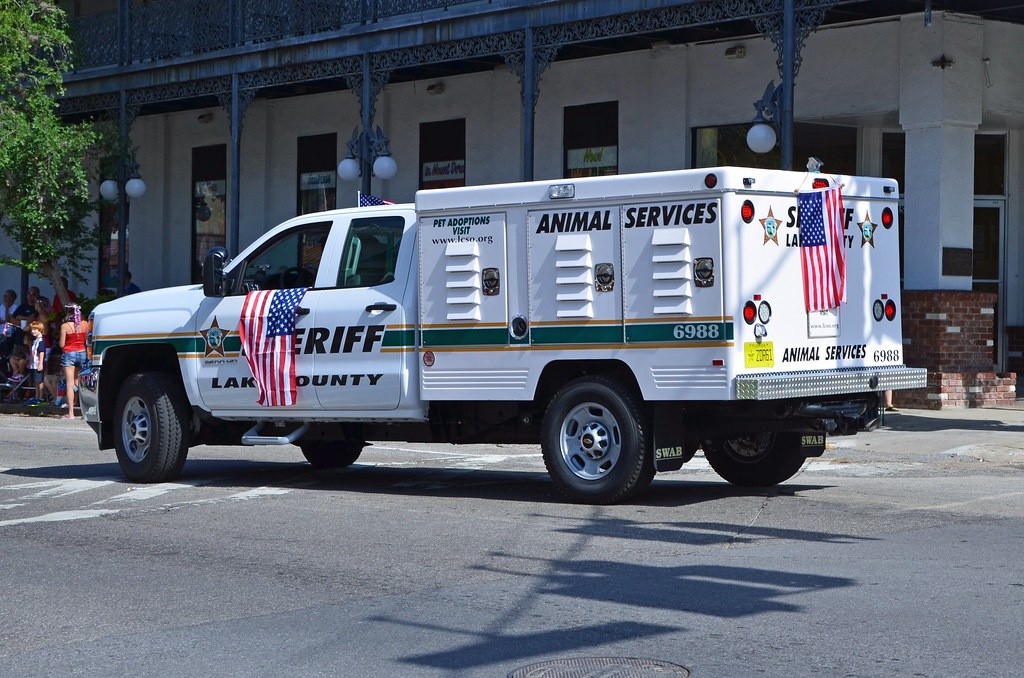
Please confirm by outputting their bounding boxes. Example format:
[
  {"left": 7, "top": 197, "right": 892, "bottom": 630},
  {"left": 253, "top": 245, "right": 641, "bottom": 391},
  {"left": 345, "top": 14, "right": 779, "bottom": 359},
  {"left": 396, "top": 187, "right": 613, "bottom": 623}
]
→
[
  {"left": 63, "top": 415, "right": 75, "bottom": 418},
  {"left": 27, "top": 396, "right": 37, "bottom": 403},
  {"left": 32, "top": 398, "right": 46, "bottom": 406},
  {"left": 7, "top": 373, "right": 25, "bottom": 382}
]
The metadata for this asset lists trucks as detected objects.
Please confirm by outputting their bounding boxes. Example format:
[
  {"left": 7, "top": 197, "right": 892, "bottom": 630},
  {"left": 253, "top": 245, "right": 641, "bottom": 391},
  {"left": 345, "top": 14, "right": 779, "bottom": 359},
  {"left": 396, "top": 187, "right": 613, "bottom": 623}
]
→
[{"left": 83, "top": 165, "right": 929, "bottom": 507}]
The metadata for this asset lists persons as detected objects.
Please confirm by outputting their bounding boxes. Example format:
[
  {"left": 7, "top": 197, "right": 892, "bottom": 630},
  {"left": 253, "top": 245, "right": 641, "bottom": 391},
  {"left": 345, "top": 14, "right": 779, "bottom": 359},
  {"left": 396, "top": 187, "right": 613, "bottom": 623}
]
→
[
  {"left": 116, "top": 270, "right": 141, "bottom": 298},
  {"left": 50, "top": 276, "right": 78, "bottom": 322},
  {"left": 59, "top": 302, "right": 89, "bottom": 419},
  {"left": 29, "top": 321, "right": 46, "bottom": 405},
  {"left": 0, "top": 286, "right": 64, "bottom": 403},
  {"left": 97, "top": 288, "right": 110, "bottom": 297},
  {"left": 884, "top": 389, "right": 899, "bottom": 412}
]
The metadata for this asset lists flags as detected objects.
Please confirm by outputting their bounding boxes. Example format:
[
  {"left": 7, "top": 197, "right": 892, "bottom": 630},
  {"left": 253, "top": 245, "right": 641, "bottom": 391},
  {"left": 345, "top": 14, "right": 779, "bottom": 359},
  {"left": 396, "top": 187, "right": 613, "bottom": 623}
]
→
[
  {"left": 796, "top": 187, "right": 847, "bottom": 314},
  {"left": 238, "top": 287, "right": 314, "bottom": 407},
  {"left": 359, "top": 194, "right": 395, "bottom": 207}
]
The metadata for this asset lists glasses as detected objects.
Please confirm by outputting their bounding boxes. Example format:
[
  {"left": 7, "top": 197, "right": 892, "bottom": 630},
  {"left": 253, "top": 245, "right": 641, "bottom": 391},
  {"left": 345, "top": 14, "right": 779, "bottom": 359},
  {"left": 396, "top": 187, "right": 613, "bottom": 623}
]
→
[{"left": 27, "top": 292, "right": 36, "bottom": 296}]
